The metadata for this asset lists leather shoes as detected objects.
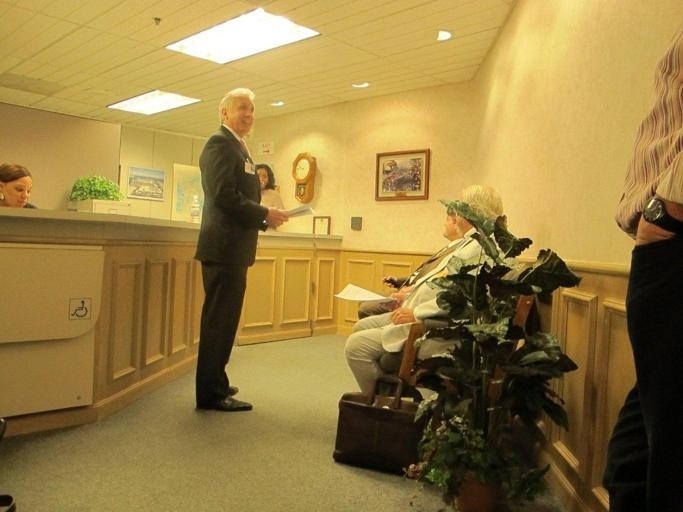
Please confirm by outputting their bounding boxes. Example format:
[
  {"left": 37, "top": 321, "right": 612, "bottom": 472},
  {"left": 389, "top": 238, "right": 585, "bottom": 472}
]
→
[
  {"left": 196, "top": 395, "right": 252, "bottom": 411},
  {"left": 225, "top": 385, "right": 239, "bottom": 396}
]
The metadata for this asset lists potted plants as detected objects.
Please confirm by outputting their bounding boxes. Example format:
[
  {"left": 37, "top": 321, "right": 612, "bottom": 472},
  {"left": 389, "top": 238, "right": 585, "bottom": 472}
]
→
[{"left": 405, "top": 194, "right": 583, "bottom": 511}]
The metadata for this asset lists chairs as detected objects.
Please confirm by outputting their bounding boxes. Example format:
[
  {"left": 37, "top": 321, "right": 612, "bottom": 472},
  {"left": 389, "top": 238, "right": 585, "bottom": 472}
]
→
[{"left": 379, "top": 292, "right": 537, "bottom": 405}]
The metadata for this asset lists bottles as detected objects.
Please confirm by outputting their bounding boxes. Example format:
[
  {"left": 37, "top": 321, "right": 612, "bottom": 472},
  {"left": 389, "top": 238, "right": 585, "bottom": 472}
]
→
[{"left": 191, "top": 196, "right": 200, "bottom": 223}]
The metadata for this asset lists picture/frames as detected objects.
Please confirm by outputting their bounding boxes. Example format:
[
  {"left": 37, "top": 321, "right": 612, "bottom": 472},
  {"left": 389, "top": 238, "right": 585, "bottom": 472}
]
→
[
  {"left": 311, "top": 216, "right": 330, "bottom": 236},
  {"left": 374, "top": 148, "right": 430, "bottom": 201},
  {"left": 125, "top": 166, "right": 166, "bottom": 202}
]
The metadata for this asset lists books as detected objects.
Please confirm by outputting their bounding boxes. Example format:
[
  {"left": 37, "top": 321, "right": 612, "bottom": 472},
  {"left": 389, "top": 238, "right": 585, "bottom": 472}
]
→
[{"left": 288, "top": 205, "right": 315, "bottom": 220}]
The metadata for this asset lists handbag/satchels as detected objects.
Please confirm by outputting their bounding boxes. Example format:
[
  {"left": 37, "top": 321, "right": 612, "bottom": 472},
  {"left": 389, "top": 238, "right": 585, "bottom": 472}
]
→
[{"left": 330, "top": 372, "right": 426, "bottom": 479}]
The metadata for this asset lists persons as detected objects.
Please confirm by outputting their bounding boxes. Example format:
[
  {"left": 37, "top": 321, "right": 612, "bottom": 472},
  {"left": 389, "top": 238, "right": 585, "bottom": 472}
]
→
[
  {"left": 191, "top": 85, "right": 290, "bottom": 413},
  {"left": 357, "top": 200, "right": 464, "bottom": 319},
  {"left": 342, "top": 182, "right": 504, "bottom": 403},
  {"left": 255, "top": 162, "right": 286, "bottom": 211},
  {"left": 0, "top": 162, "right": 41, "bottom": 210},
  {"left": 600, "top": 16, "right": 682, "bottom": 511}
]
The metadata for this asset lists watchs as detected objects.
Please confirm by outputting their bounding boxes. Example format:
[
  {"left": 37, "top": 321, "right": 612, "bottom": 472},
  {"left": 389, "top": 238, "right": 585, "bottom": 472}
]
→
[{"left": 641, "top": 196, "right": 682, "bottom": 236}]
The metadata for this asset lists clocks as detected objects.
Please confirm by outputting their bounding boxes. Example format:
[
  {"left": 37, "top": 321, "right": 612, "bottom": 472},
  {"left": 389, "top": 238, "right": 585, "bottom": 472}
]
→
[{"left": 291, "top": 152, "right": 316, "bottom": 204}]
